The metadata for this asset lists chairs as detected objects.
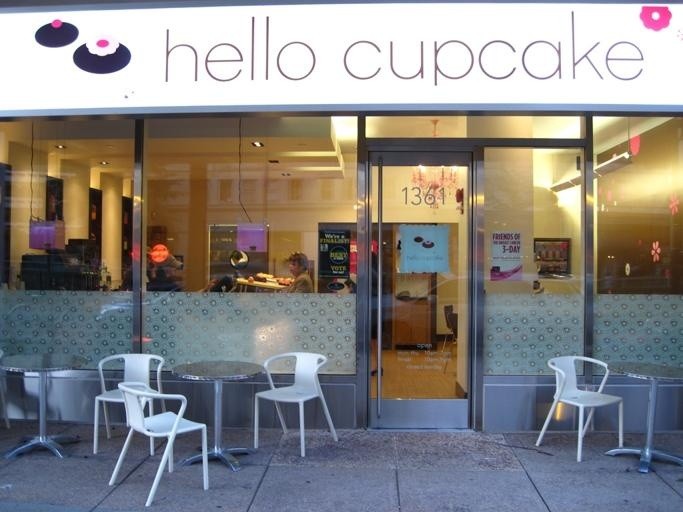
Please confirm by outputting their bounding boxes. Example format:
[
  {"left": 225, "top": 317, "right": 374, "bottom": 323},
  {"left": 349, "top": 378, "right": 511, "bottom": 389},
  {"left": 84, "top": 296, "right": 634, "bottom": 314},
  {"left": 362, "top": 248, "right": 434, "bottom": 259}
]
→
[
  {"left": 93, "top": 353, "right": 168, "bottom": 455},
  {"left": 0, "top": 349, "right": 11, "bottom": 430},
  {"left": 108, "top": 381, "right": 209, "bottom": 507},
  {"left": 534, "top": 355, "right": 624, "bottom": 462},
  {"left": 443, "top": 313, "right": 458, "bottom": 374},
  {"left": 254, "top": 352, "right": 338, "bottom": 457},
  {"left": 441, "top": 305, "right": 454, "bottom": 352}
]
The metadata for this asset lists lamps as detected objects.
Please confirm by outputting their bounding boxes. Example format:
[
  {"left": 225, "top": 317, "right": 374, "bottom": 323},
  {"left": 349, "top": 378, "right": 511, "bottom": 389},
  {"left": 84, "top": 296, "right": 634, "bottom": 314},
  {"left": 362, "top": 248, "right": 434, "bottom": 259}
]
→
[
  {"left": 409, "top": 120, "right": 458, "bottom": 210},
  {"left": 550, "top": 117, "right": 633, "bottom": 191},
  {"left": 150, "top": 244, "right": 169, "bottom": 279}
]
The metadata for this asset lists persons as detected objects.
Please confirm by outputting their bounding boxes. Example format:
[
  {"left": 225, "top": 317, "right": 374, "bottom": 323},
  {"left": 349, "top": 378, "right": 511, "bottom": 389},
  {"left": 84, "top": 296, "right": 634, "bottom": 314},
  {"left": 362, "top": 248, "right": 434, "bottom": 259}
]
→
[
  {"left": 146, "top": 251, "right": 219, "bottom": 296},
  {"left": 367, "top": 249, "right": 386, "bottom": 378},
  {"left": 281, "top": 253, "right": 312, "bottom": 292}
]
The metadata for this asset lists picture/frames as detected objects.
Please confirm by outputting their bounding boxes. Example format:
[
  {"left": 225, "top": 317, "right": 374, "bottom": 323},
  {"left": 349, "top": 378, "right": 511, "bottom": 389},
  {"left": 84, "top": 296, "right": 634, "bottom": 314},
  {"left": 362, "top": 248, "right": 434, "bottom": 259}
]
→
[
  {"left": 534, "top": 238, "right": 571, "bottom": 275},
  {"left": 174, "top": 255, "right": 183, "bottom": 270}
]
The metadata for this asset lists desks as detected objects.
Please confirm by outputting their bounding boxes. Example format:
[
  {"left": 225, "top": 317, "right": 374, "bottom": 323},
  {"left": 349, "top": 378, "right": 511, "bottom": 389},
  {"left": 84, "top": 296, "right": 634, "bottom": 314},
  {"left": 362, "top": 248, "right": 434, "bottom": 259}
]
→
[
  {"left": 0, "top": 354, "right": 88, "bottom": 459},
  {"left": 603, "top": 361, "right": 683, "bottom": 473},
  {"left": 171, "top": 361, "right": 264, "bottom": 472}
]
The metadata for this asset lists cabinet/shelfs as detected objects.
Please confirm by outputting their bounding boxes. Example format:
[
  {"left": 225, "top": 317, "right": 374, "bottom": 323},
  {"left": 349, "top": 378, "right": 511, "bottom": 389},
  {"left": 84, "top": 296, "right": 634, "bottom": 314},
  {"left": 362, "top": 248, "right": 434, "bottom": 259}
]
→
[
  {"left": 393, "top": 225, "right": 437, "bottom": 351},
  {"left": 21, "top": 254, "right": 99, "bottom": 290}
]
